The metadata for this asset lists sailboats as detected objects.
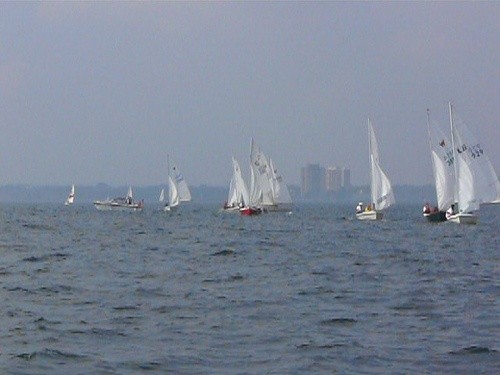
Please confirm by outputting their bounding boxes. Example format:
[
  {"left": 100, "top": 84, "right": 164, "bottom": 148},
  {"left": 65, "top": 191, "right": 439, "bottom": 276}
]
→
[
  {"left": 356, "top": 117, "right": 395, "bottom": 221},
  {"left": 221, "top": 138, "right": 294, "bottom": 216},
  {"left": 155, "top": 166, "right": 192, "bottom": 214},
  {"left": 446, "top": 101, "right": 500, "bottom": 224},
  {"left": 425, "top": 107, "right": 456, "bottom": 222},
  {"left": 64, "top": 185, "right": 75, "bottom": 207}
]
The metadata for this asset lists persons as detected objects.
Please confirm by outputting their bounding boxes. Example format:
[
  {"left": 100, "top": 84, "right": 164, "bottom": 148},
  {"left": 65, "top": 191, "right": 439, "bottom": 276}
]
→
[
  {"left": 356, "top": 202, "right": 364, "bottom": 213},
  {"left": 423, "top": 202, "right": 431, "bottom": 217},
  {"left": 365, "top": 204, "right": 371, "bottom": 212},
  {"left": 446, "top": 204, "right": 455, "bottom": 219}
]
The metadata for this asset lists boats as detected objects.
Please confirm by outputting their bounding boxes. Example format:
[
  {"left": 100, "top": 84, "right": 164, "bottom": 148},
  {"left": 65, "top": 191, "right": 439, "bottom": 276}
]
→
[{"left": 93, "top": 185, "right": 144, "bottom": 213}]
